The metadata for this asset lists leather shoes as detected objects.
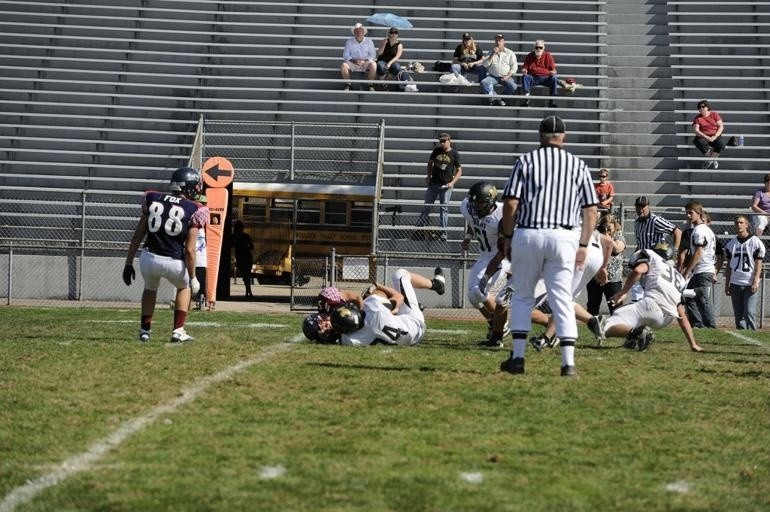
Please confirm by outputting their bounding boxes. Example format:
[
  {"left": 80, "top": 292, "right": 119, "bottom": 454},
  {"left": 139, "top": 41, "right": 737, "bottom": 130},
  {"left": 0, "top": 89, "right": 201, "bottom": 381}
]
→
[
  {"left": 523, "top": 100, "right": 529, "bottom": 107},
  {"left": 550, "top": 100, "right": 557, "bottom": 107}
]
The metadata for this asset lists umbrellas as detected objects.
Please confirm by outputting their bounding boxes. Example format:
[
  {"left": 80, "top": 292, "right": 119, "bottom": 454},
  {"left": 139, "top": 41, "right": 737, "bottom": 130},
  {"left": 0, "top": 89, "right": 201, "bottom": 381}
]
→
[{"left": 366, "top": 12, "right": 414, "bottom": 40}]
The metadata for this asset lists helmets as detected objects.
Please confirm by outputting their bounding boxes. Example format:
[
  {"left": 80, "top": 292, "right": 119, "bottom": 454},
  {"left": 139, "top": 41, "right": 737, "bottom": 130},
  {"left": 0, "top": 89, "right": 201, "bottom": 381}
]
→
[
  {"left": 651, "top": 243, "right": 673, "bottom": 260},
  {"left": 467, "top": 183, "right": 497, "bottom": 218},
  {"left": 303, "top": 313, "right": 328, "bottom": 339},
  {"left": 171, "top": 168, "right": 200, "bottom": 200},
  {"left": 330, "top": 303, "right": 366, "bottom": 334}
]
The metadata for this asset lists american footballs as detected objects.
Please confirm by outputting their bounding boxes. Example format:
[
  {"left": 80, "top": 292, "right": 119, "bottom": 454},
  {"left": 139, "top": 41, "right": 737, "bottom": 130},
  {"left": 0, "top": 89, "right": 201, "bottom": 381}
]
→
[{"left": 363, "top": 290, "right": 389, "bottom": 298}]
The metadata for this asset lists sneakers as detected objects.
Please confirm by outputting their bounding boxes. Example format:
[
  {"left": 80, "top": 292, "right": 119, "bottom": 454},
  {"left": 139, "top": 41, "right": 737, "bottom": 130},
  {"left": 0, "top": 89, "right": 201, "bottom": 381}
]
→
[
  {"left": 435, "top": 267, "right": 444, "bottom": 294},
  {"left": 714, "top": 161, "right": 719, "bottom": 169},
  {"left": 637, "top": 328, "right": 654, "bottom": 352},
  {"left": 501, "top": 350, "right": 524, "bottom": 373},
  {"left": 478, "top": 341, "right": 503, "bottom": 348},
  {"left": 441, "top": 231, "right": 447, "bottom": 240},
  {"left": 625, "top": 339, "right": 639, "bottom": 348},
  {"left": 368, "top": 84, "right": 374, "bottom": 91},
  {"left": 172, "top": 327, "right": 193, "bottom": 342},
  {"left": 140, "top": 328, "right": 151, "bottom": 341},
  {"left": 490, "top": 98, "right": 499, "bottom": 106},
  {"left": 588, "top": 315, "right": 605, "bottom": 347},
  {"left": 704, "top": 160, "right": 713, "bottom": 169},
  {"left": 694, "top": 287, "right": 704, "bottom": 313},
  {"left": 344, "top": 83, "right": 351, "bottom": 90},
  {"left": 541, "top": 334, "right": 559, "bottom": 348},
  {"left": 530, "top": 335, "right": 548, "bottom": 351},
  {"left": 498, "top": 99, "right": 506, "bottom": 106},
  {"left": 561, "top": 365, "right": 574, "bottom": 375},
  {"left": 487, "top": 325, "right": 510, "bottom": 338}
]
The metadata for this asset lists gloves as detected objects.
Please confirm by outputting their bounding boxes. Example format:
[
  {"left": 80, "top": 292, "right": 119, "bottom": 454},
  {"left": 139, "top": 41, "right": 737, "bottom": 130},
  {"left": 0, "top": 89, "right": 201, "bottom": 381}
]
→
[
  {"left": 123, "top": 264, "right": 135, "bottom": 285},
  {"left": 191, "top": 277, "right": 200, "bottom": 294}
]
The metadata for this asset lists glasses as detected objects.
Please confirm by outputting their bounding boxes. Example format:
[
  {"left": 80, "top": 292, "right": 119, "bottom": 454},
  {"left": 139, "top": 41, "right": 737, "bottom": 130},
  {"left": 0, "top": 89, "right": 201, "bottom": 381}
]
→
[
  {"left": 535, "top": 46, "right": 544, "bottom": 50},
  {"left": 599, "top": 175, "right": 606, "bottom": 178},
  {"left": 698, "top": 106, "right": 708, "bottom": 109},
  {"left": 390, "top": 31, "right": 398, "bottom": 34},
  {"left": 440, "top": 141, "right": 446, "bottom": 143}
]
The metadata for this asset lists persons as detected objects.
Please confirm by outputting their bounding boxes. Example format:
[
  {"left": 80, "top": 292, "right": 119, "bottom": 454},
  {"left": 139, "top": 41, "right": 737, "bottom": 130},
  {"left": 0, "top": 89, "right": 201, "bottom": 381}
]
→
[
  {"left": 377, "top": 27, "right": 403, "bottom": 90},
  {"left": 302, "top": 283, "right": 423, "bottom": 343},
  {"left": 123, "top": 167, "right": 202, "bottom": 341},
  {"left": 478, "top": 218, "right": 511, "bottom": 348},
  {"left": 679, "top": 211, "right": 724, "bottom": 314},
  {"left": 628, "top": 196, "right": 683, "bottom": 305},
  {"left": 460, "top": 180, "right": 511, "bottom": 339},
  {"left": 331, "top": 266, "right": 444, "bottom": 347},
  {"left": 682, "top": 202, "right": 717, "bottom": 329},
  {"left": 452, "top": 33, "right": 489, "bottom": 94},
  {"left": 529, "top": 216, "right": 605, "bottom": 352},
  {"left": 411, "top": 132, "right": 462, "bottom": 241},
  {"left": 341, "top": 23, "right": 377, "bottom": 90},
  {"left": 752, "top": 173, "right": 770, "bottom": 236},
  {"left": 602, "top": 241, "right": 706, "bottom": 353},
  {"left": 500, "top": 115, "right": 598, "bottom": 375},
  {"left": 692, "top": 100, "right": 727, "bottom": 169},
  {"left": 596, "top": 169, "right": 616, "bottom": 207},
  {"left": 480, "top": 34, "right": 518, "bottom": 106},
  {"left": 230, "top": 221, "right": 254, "bottom": 297},
  {"left": 520, "top": 40, "right": 560, "bottom": 108},
  {"left": 724, "top": 215, "right": 766, "bottom": 330},
  {"left": 587, "top": 213, "right": 627, "bottom": 340}
]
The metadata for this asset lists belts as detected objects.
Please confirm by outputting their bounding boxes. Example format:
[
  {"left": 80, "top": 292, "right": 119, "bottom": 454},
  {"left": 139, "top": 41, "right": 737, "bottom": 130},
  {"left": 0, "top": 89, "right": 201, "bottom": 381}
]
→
[{"left": 592, "top": 243, "right": 598, "bottom": 248}]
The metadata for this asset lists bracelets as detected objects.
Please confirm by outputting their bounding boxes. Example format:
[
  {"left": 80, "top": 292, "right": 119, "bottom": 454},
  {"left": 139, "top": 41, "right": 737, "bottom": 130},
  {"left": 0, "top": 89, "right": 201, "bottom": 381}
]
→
[
  {"left": 579, "top": 240, "right": 588, "bottom": 248},
  {"left": 504, "top": 233, "right": 514, "bottom": 239}
]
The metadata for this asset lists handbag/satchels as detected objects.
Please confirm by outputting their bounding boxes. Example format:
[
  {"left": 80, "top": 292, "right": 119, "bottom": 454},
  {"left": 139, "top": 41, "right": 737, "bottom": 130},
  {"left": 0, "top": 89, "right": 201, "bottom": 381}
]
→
[
  {"left": 397, "top": 69, "right": 413, "bottom": 89},
  {"left": 434, "top": 63, "right": 450, "bottom": 71}
]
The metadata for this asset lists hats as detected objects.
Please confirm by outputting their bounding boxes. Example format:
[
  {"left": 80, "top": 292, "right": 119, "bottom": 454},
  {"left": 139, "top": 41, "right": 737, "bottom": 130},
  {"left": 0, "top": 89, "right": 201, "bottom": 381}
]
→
[
  {"left": 567, "top": 78, "right": 576, "bottom": 83},
  {"left": 539, "top": 116, "right": 566, "bottom": 133},
  {"left": 439, "top": 133, "right": 451, "bottom": 141},
  {"left": 389, "top": 27, "right": 400, "bottom": 32},
  {"left": 635, "top": 196, "right": 648, "bottom": 206},
  {"left": 352, "top": 23, "right": 367, "bottom": 35},
  {"left": 495, "top": 34, "right": 504, "bottom": 38},
  {"left": 463, "top": 32, "right": 471, "bottom": 38}
]
